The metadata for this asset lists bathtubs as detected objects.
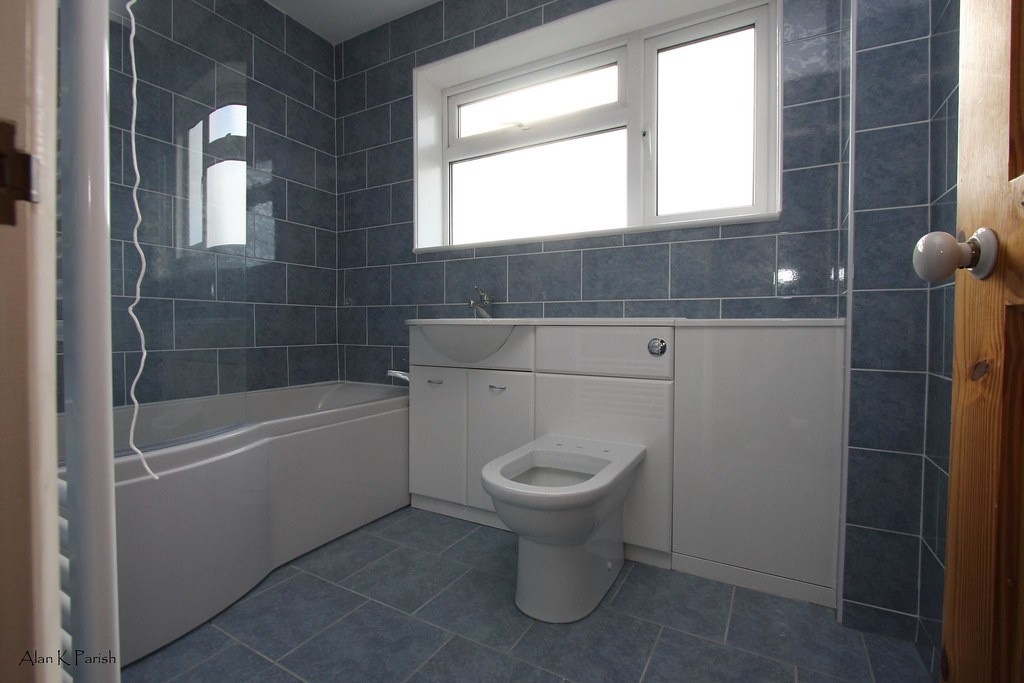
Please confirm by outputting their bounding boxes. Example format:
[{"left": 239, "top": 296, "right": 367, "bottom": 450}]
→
[{"left": 56, "top": 379, "right": 411, "bottom": 674}]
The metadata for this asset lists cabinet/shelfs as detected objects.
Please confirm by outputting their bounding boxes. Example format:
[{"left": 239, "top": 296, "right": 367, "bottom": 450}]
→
[
  {"left": 671, "top": 326, "right": 842, "bottom": 609},
  {"left": 407, "top": 365, "right": 538, "bottom": 532},
  {"left": 532, "top": 324, "right": 671, "bottom": 570}
]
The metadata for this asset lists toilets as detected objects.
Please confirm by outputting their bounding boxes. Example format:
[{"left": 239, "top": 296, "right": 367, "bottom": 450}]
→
[{"left": 480, "top": 433, "right": 649, "bottom": 624}]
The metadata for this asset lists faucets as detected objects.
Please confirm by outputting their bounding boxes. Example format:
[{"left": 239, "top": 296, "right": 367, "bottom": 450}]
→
[{"left": 468, "top": 285, "right": 494, "bottom": 318}]
